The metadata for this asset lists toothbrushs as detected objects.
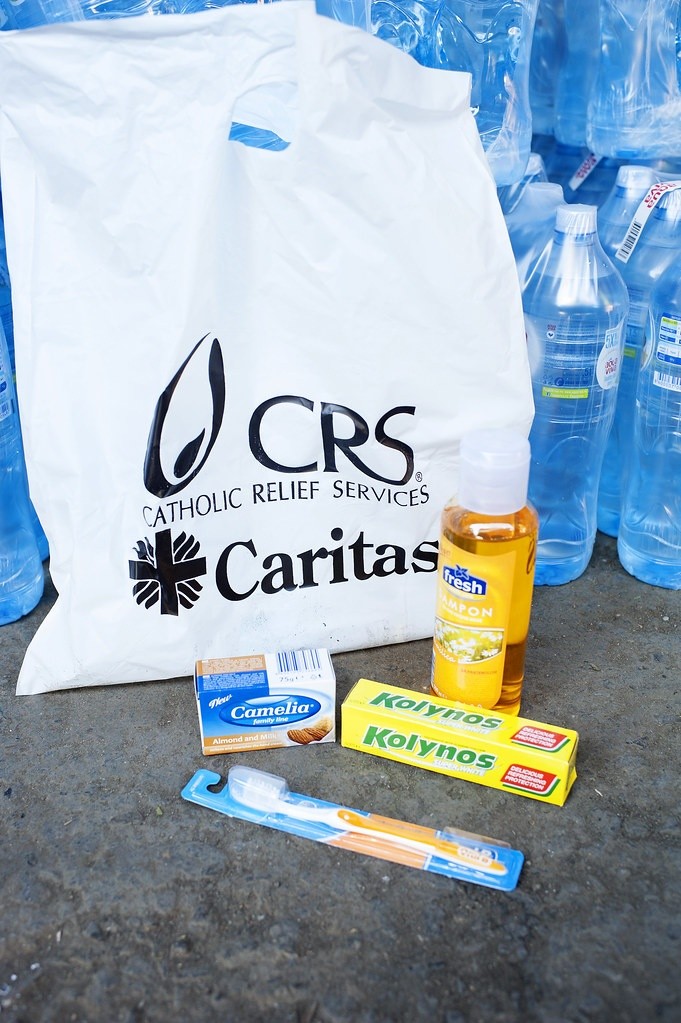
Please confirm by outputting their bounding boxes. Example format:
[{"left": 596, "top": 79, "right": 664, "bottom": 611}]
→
[{"left": 179, "top": 763, "right": 526, "bottom": 895}]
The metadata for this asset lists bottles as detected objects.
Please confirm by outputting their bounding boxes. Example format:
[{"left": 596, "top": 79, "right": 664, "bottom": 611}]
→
[
  {"left": 317, "top": 1, "right": 681, "bottom": 592},
  {"left": 429, "top": 428, "right": 541, "bottom": 714}
]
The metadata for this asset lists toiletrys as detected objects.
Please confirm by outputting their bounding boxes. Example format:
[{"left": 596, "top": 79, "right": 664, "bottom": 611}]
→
[{"left": 190, "top": 425, "right": 580, "bottom": 807}]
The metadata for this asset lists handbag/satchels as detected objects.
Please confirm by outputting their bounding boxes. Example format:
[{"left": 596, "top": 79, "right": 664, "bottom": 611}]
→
[{"left": 1, "top": 4, "right": 534, "bottom": 696}]
